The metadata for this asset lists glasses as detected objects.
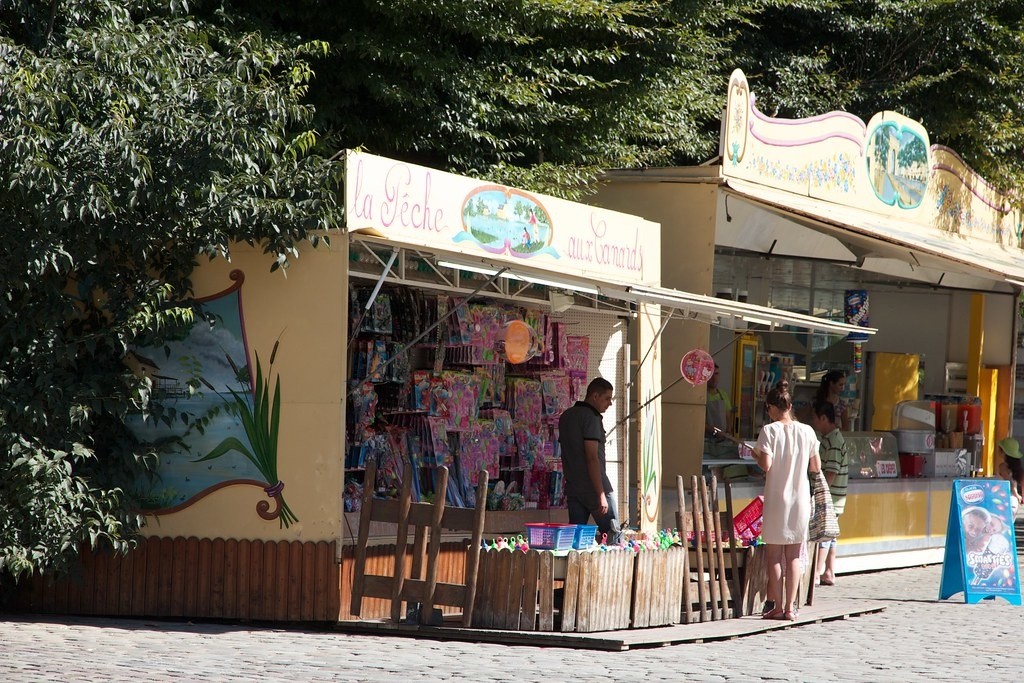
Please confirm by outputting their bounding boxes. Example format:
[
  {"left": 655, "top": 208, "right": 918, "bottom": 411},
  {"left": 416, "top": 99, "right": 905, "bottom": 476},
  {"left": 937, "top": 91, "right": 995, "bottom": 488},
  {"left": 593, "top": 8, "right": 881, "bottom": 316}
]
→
[{"left": 765, "top": 405, "right": 772, "bottom": 415}]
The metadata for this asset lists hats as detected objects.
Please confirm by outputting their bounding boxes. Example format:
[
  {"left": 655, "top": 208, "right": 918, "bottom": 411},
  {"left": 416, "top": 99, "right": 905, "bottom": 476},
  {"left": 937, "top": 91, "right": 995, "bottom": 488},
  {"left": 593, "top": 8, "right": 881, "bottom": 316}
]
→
[{"left": 997, "top": 438, "right": 1023, "bottom": 458}]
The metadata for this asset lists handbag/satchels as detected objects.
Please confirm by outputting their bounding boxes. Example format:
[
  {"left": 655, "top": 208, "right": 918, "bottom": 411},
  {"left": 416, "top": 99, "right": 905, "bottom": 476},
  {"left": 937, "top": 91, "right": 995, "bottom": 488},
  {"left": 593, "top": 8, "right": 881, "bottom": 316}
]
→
[{"left": 808, "top": 467, "right": 841, "bottom": 543}]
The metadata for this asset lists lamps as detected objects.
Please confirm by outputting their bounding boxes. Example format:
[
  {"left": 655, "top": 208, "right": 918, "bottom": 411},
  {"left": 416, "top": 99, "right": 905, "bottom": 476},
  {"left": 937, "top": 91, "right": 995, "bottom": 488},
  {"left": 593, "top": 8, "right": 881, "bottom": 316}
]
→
[{"left": 549, "top": 289, "right": 574, "bottom": 313}]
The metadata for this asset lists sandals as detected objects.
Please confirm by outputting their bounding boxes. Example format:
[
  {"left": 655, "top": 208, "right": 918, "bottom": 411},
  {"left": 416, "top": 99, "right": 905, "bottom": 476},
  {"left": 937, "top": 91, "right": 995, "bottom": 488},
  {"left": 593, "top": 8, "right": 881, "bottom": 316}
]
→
[
  {"left": 763, "top": 609, "right": 785, "bottom": 620},
  {"left": 785, "top": 607, "right": 799, "bottom": 620}
]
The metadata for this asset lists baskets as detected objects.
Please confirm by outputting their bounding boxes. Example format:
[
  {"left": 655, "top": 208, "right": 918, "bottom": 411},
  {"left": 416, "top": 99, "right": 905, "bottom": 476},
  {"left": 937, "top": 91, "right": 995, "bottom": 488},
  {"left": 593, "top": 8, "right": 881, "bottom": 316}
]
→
[
  {"left": 524, "top": 522, "right": 577, "bottom": 550},
  {"left": 572, "top": 525, "right": 598, "bottom": 549}
]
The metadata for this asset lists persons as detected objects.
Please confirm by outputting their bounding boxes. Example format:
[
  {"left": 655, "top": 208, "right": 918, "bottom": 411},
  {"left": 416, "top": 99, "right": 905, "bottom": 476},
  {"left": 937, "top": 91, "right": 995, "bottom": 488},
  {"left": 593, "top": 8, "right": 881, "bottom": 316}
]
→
[
  {"left": 558, "top": 378, "right": 621, "bottom": 545},
  {"left": 980, "top": 514, "right": 1009, "bottom": 536},
  {"left": 994, "top": 437, "right": 1024, "bottom": 523},
  {"left": 814, "top": 371, "right": 852, "bottom": 431},
  {"left": 703, "top": 362, "right": 737, "bottom": 484},
  {"left": 750, "top": 379, "right": 822, "bottom": 618},
  {"left": 811, "top": 399, "right": 849, "bottom": 584},
  {"left": 960, "top": 506, "right": 993, "bottom": 553}
]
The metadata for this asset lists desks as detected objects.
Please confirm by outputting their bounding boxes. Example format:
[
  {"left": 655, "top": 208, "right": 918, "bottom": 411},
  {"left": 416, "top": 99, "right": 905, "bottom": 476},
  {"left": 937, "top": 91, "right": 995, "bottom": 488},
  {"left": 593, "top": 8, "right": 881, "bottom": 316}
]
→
[{"left": 555, "top": 542, "right": 819, "bottom": 616}]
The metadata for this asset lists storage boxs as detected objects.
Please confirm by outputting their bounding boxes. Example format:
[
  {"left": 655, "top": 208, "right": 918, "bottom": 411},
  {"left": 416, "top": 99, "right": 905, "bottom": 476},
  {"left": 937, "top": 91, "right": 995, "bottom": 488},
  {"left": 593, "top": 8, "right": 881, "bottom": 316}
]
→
[
  {"left": 572, "top": 525, "right": 599, "bottom": 549},
  {"left": 524, "top": 522, "right": 576, "bottom": 550}
]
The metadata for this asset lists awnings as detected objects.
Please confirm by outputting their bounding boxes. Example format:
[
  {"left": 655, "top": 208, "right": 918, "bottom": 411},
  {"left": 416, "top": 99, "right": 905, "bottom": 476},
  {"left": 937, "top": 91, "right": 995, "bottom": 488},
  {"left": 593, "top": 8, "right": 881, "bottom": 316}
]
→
[
  {"left": 348, "top": 231, "right": 880, "bottom": 339},
  {"left": 722, "top": 175, "right": 1023, "bottom": 291}
]
